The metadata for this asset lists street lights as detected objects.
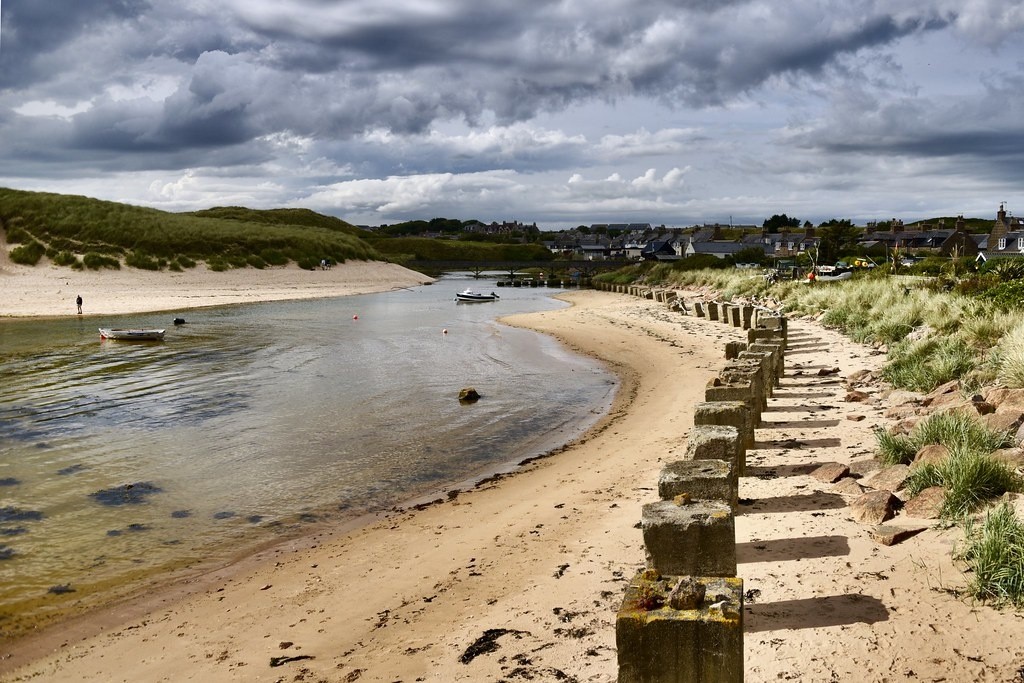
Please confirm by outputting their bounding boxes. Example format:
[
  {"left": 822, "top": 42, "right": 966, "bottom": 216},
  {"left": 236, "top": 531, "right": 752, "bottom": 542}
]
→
[
  {"left": 957, "top": 231, "right": 965, "bottom": 258},
  {"left": 882, "top": 241, "right": 888, "bottom": 264}
]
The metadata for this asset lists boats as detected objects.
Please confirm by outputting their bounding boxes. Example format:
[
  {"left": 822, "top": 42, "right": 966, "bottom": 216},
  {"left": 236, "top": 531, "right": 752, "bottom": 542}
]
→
[
  {"left": 455, "top": 287, "right": 500, "bottom": 303},
  {"left": 98, "top": 326, "right": 166, "bottom": 340}
]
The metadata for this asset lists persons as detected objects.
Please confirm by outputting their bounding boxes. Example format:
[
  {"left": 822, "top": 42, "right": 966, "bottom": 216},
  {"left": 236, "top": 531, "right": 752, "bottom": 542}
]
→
[{"left": 76, "top": 295, "right": 83, "bottom": 314}]
